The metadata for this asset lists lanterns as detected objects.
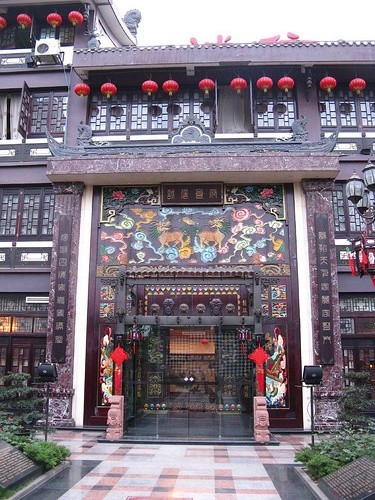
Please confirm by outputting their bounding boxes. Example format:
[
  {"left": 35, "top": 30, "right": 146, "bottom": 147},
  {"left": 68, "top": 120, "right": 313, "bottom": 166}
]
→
[
  {"left": 201, "top": 339, "right": 208, "bottom": 344},
  {"left": 309, "top": 94, "right": 375, "bottom": 118},
  {"left": 256, "top": 77, "right": 273, "bottom": 92},
  {"left": 148, "top": 102, "right": 211, "bottom": 117},
  {"left": 230, "top": 78, "right": 247, "bottom": 94},
  {"left": 198, "top": 78, "right": 215, "bottom": 95},
  {"left": 277, "top": 77, "right": 294, "bottom": 92},
  {"left": 100, "top": 83, "right": 117, "bottom": 99},
  {"left": 349, "top": 78, "right": 366, "bottom": 94},
  {"left": 0, "top": 16, "right": 7, "bottom": 30},
  {"left": 68, "top": 11, "right": 84, "bottom": 26},
  {"left": 141, "top": 80, "right": 158, "bottom": 96},
  {"left": 47, "top": 13, "right": 63, "bottom": 27},
  {"left": 89, "top": 104, "right": 124, "bottom": 117},
  {"left": 74, "top": 83, "right": 90, "bottom": 98},
  {"left": 320, "top": 77, "right": 336, "bottom": 92},
  {"left": 17, "top": 13, "right": 31, "bottom": 29},
  {"left": 256, "top": 103, "right": 286, "bottom": 117},
  {"left": 162, "top": 80, "right": 179, "bottom": 97}
]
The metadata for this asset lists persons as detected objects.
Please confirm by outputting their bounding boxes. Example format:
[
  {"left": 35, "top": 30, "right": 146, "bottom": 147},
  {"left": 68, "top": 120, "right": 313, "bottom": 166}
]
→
[{"left": 197, "top": 374, "right": 208, "bottom": 402}]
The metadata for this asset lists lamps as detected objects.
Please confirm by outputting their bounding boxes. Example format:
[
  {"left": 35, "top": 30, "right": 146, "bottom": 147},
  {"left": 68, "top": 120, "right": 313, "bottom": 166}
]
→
[
  {"left": 302, "top": 365, "right": 323, "bottom": 385},
  {"left": 34, "top": 362, "right": 58, "bottom": 383}
]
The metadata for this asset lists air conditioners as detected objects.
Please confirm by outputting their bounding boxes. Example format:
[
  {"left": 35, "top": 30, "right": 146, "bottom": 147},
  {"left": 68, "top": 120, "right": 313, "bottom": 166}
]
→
[{"left": 34, "top": 38, "right": 61, "bottom": 62}]
